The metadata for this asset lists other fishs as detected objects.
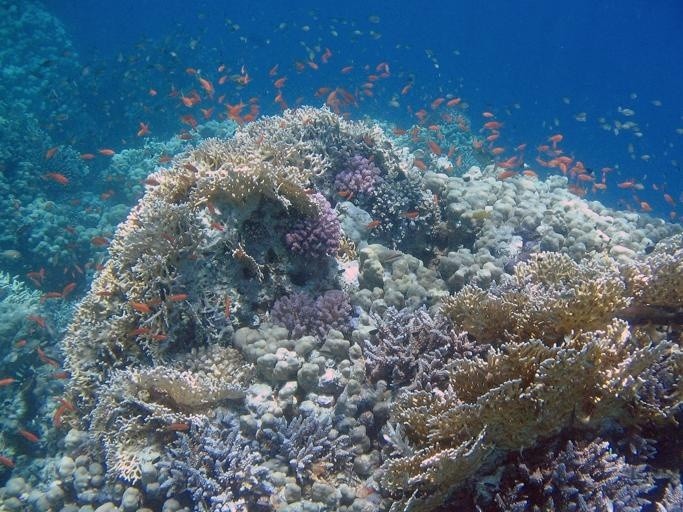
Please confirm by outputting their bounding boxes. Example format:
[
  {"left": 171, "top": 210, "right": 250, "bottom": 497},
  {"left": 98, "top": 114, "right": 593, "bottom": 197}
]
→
[{"left": 1, "top": 11, "right": 683, "bottom": 471}]
